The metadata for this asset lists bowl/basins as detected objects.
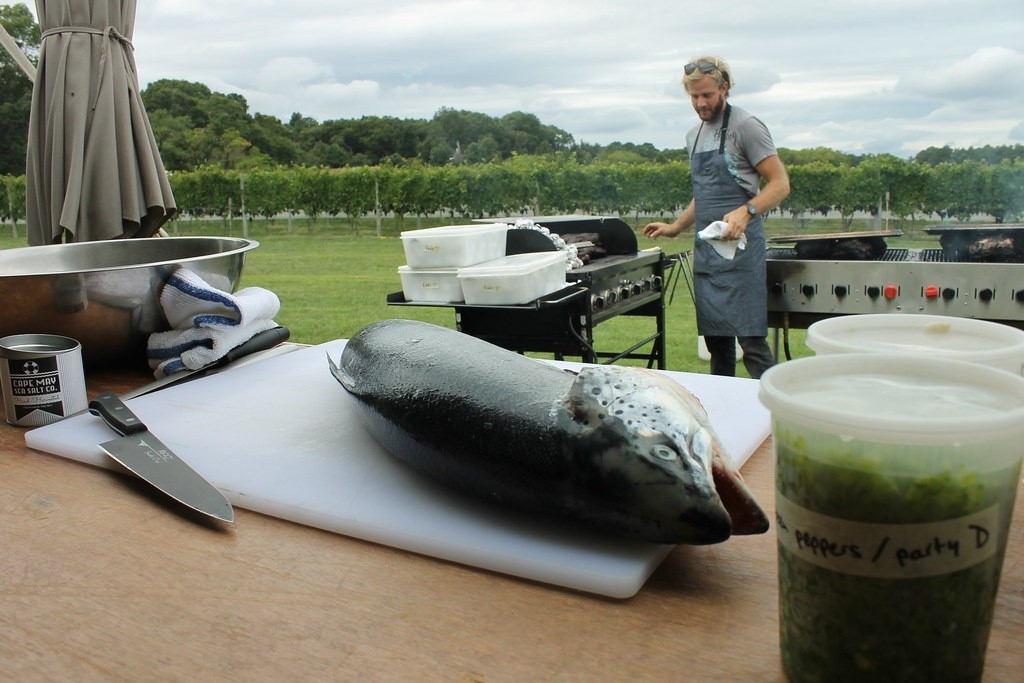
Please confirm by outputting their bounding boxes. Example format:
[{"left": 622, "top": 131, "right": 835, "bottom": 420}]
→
[{"left": 0, "top": 237, "right": 259, "bottom": 358}]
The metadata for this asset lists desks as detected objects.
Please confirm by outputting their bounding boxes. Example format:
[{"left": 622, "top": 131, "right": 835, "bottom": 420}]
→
[{"left": 0, "top": 365, "right": 1024, "bottom": 683}]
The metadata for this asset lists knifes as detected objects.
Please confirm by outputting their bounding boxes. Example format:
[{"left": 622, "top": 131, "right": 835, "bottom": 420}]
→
[
  {"left": 89, "top": 393, "right": 234, "bottom": 523},
  {"left": 53, "top": 326, "right": 290, "bottom": 425}
]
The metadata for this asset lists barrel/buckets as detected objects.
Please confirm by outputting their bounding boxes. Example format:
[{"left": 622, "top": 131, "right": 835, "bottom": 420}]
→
[{"left": 696, "top": 336, "right": 744, "bottom": 361}]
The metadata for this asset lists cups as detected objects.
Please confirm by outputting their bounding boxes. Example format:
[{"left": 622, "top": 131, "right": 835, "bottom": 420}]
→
[
  {"left": 805, "top": 314, "right": 1024, "bottom": 380},
  {"left": 759, "top": 354, "right": 1024, "bottom": 682}
]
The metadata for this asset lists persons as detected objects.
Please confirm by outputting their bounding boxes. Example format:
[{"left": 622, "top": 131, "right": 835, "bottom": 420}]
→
[{"left": 643, "top": 55, "right": 790, "bottom": 379}]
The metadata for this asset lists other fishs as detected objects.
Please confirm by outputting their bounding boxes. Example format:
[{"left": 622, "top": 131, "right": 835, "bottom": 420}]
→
[{"left": 325, "top": 319, "right": 771, "bottom": 545}]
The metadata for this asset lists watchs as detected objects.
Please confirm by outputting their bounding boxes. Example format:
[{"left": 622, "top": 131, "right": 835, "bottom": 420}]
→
[{"left": 744, "top": 201, "right": 757, "bottom": 217}]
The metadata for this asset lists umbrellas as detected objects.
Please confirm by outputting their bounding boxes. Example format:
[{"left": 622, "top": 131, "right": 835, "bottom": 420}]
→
[{"left": 25, "top": 0, "right": 178, "bottom": 246}]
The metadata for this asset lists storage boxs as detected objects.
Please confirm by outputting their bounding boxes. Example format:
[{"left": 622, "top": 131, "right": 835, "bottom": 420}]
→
[
  {"left": 398, "top": 222, "right": 509, "bottom": 271},
  {"left": 459, "top": 251, "right": 568, "bottom": 304},
  {"left": 397, "top": 265, "right": 464, "bottom": 303}
]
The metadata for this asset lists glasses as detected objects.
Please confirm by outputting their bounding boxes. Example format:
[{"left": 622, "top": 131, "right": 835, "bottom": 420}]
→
[{"left": 684, "top": 62, "right": 722, "bottom": 76}]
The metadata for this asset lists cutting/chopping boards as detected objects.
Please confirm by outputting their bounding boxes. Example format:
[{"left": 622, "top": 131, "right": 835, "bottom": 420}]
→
[{"left": 25, "top": 340, "right": 772, "bottom": 599}]
